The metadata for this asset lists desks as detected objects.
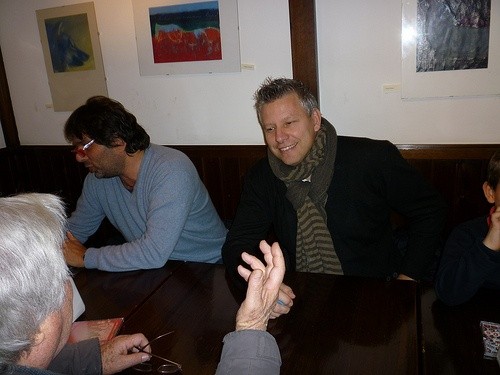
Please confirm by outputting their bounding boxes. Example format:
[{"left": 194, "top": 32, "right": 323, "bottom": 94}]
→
[
  {"left": 418, "top": 280, "right": 500, "bottom": 375},
  {"left": 71, "top": 259, "right": 420, "bottom": 375}
]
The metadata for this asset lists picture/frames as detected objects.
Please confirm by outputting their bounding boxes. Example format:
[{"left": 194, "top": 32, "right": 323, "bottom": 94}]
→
[
  {"left": 131, "top": 0, "right": 241, "bottom": 77},
  {"left": 35, "top": 1, "right": 110, "bottom": 113},
  {"left": 399, "top": 0, "right": 500, "bottom": 100}
]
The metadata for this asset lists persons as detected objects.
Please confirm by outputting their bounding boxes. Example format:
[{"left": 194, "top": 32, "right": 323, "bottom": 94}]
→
[
  {"left": 220, "top": 75, "right": 419, "bottom": 345},
  {"left": 0, "top": 192, "right": 285, "bottom": 375},
  {"left": 435, "top": 151, "right": 500, "bottom": 305},
  {"left": 63, "top": 95, "right": 228, "bottom": 272}
]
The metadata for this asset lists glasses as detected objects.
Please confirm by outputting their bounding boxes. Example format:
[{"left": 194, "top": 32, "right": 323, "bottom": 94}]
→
[
  {"left": 71, "top": 139, "right": 95, "bottom": 157},
  {"left": 133, "top": 331, "right": 183, "bottom": 374}
]
